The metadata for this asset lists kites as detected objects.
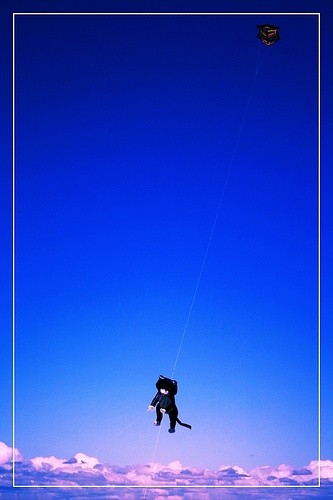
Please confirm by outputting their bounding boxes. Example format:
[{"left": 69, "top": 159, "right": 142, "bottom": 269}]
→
[
  {"left": 255, "top": 24, "right": 280, "bottom": 47},
  {"left": 146, "top": 374, "right": 191, "bottom": 434}
]
